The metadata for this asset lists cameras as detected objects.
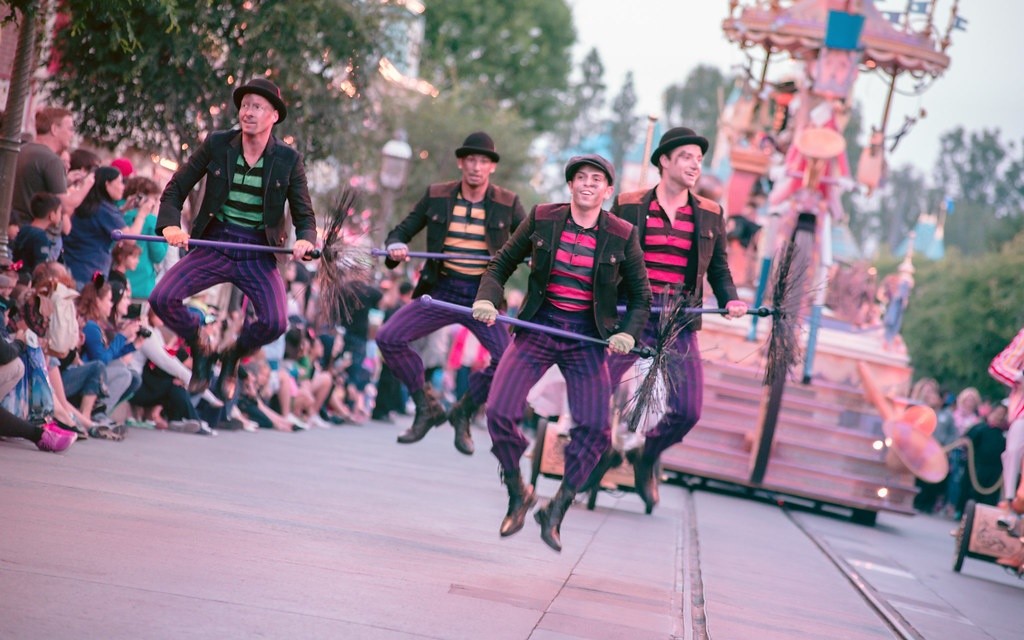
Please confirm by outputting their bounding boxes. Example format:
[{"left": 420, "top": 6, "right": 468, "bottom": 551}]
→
[{"left": 136, "top": 325, "right": 152, "bottom": 337}]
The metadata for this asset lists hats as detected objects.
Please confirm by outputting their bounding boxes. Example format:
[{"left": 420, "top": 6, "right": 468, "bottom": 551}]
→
[
  {"left": 651, "top": 127, "right": 709, "bottom": 167},
  {"left": 565, "top": 154, "right": 615, "bottom": 185},
  {"left": 233, "top": 78, "right": 288, "bottom": 126},
  {"left": 110, "top": 280, "right": 124, "bottom": 302},
  {"left": 455, "top": 132, "right": 500, "bottom": 163}
]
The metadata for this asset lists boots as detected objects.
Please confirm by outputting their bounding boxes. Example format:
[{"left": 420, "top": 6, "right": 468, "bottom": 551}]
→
[
  {"left": 216, "top": 339, "right": 239, "bottom": 404},
  {"left": 578, "top": 450, "right": 622, "bottom": 494},
  {"left": 533, "top": 483, "right": 575, "bottom": 553},
  {"left": 624, "top": 439, "right": 664, "bottom": 506},
  {"left": 447, "top": 391, "right": 480, "bottom": 455},
  {"left": 398, "top": 384, "right": 447, "bottom": 445},
  {"left": 188, "top": 324, "right": 214, "bottom": 396},
  {"left": 499, "top": 468, "right": 538, "bottom": 538}
]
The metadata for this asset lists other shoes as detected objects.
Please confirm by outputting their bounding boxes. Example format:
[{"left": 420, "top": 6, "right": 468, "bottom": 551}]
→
[
  {"left": 950, "top": 527, "right": 963, "bottom": 537},
  {"left": 997, "top": 516, "right": 1022, "bottom": 539},
  {"left": 246, "top": 412, "right": 395, "bottom": 433},
  {"left": 912, "top": 503, "right": 964, "bottom": 522},
  {"left": 28, "top": 416, "right": 130, "bottom": 441},
  {"left": 124, "top": 414, "right": 259, "bottom": 436}
]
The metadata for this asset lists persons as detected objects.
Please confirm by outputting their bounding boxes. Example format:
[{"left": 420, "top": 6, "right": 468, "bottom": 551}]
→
[
  {"left": 0, "top": 78, "right": 527, "bottom": 453},
  {"left": 912, "top": 321, "right": 1024, "bottom": 517},
  {"left": 576, "top": 127, "right": 749, "bottom": 508},
  {"left": 471, "top": 154, "right": 653, "bottom": 551}
]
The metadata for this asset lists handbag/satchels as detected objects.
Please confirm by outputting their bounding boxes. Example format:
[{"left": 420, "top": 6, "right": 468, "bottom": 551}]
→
[{"left": 25, "top": 293, "right": 53, "bottom": 337}]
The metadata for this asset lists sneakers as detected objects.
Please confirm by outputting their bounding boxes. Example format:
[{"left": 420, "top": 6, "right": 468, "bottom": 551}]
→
[{"left": 35, "top": 423, "right": 78, "bottom": 452}]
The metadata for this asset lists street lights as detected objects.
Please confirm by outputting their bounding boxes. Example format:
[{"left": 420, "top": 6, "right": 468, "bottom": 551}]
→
[{"left": 368, "top": 129, "right": 412, "bottom": 291}]
